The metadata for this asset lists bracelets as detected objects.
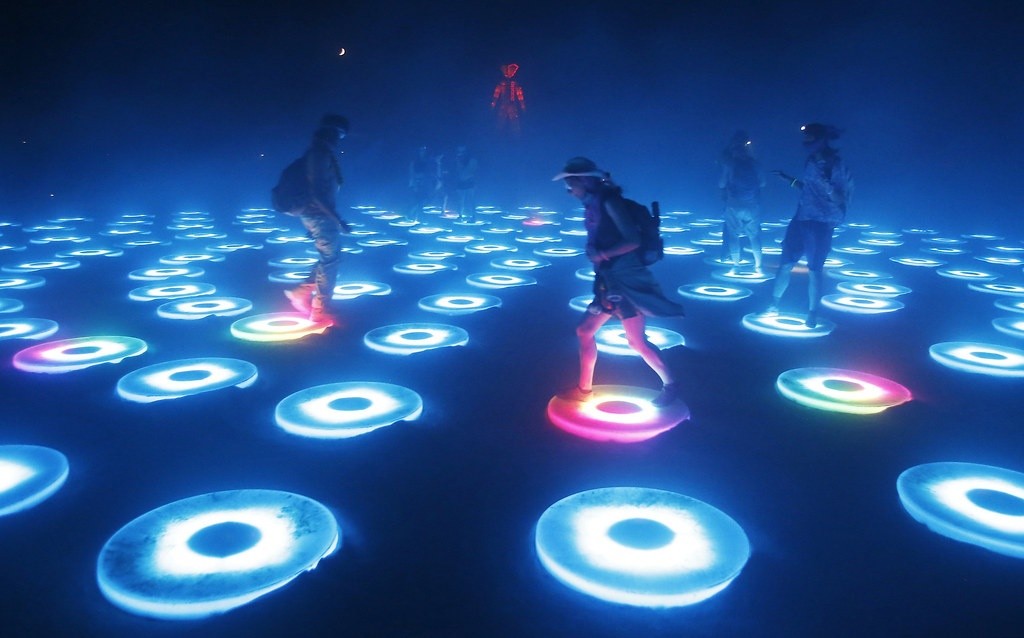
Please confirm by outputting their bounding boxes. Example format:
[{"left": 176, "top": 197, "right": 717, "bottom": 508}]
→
[
  {"left": 598, "top": 250, "right": 610, "bottom": 261},
  {"left": 790, "top": 178, "right": 798, "bottom": 189}
]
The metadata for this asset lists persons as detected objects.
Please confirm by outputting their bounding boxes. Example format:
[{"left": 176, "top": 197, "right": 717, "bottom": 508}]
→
[
  {"left": 715, "top": 130, "right": 771, "bottom": 278},
  {"left": 281, "top": 113, "right": 352, "bottom": 332},
  {"left": 409, "top": 144, "right": 479, "bottom": 223},
  {"left": 758, "top": 124, "right": 855, "bottom": 327},
  {"left": 553, "top": 156, "right": 687, "bottom": 410}
]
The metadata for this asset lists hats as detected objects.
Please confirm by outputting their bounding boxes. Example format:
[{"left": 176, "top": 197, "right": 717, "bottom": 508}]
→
[
  {"left": 800, "top": 123, "right": 838, "bottom": 140},
  {"left": 552, "top": 156, "right": 607, "bottom": 182}
]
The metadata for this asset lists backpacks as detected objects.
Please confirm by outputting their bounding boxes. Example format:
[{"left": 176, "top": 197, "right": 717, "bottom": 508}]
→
[
  {"left": 273, "top": 146, "right": 321, "bottom": 216},
  {"left": 601, "top": 192, "right": 664, "bottom": 267}
]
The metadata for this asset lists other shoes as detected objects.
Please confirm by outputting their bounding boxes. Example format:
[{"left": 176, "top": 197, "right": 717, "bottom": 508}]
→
[
  {"left": 806, "top": 311, "right": 817, "bottom": 328},
  {"left": 282, "top": 284, "right": 311, "bottom": 314},
  {"left": 724, "top": 266, "right": 740, "bottom": 276},
  {"left": 755, "top": 303, "right": 779, "bottom": 317},
  {"left": 556, "top": 387, "right": 593, "bottom": 402},
  {"left": 752, "top": 267, "right": 761, "bottom": 274},
  {"left": 651, "top": 381, "right": 684, "bottom": 408},
  {"left": 310, "top": 308, "right": 342, "bottom": 322}
]
